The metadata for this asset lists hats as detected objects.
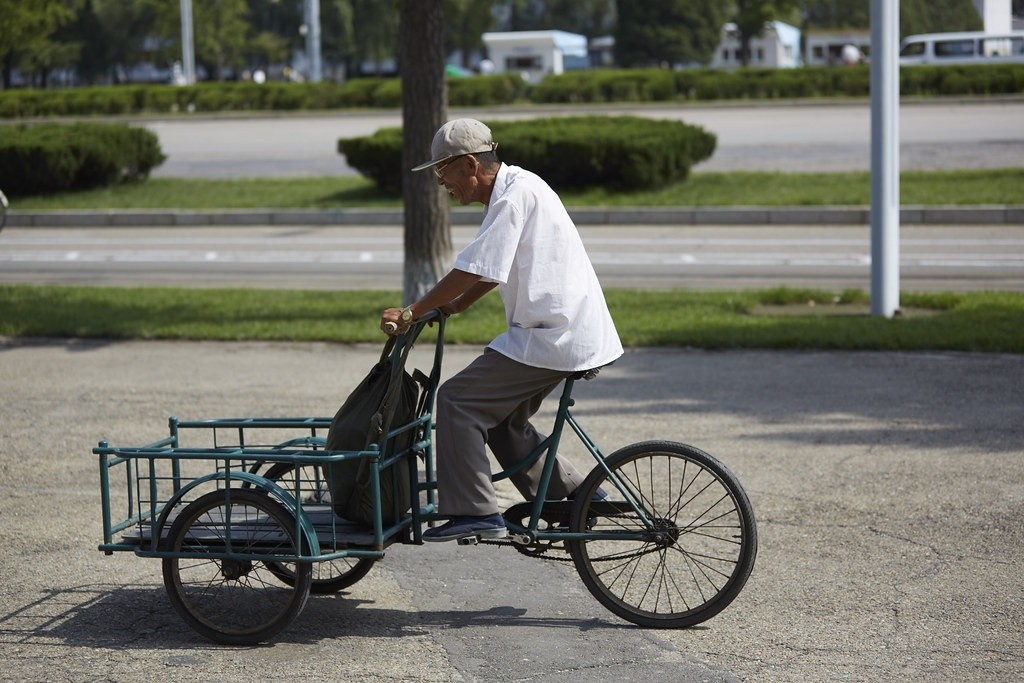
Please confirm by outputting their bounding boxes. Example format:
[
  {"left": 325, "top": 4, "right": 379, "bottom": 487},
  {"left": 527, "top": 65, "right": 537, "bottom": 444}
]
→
[{"left": 410, "top": 118, "right": 499, "bottom": 172}]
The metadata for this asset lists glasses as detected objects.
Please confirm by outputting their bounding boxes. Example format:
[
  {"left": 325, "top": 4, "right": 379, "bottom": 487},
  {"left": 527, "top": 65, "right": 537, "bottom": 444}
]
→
[{"left": 433, "top": 157, "right": 461, "bottom": 179}]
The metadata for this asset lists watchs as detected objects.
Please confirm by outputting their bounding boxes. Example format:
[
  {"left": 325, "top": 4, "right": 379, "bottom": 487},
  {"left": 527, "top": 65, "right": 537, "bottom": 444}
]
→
[{"left": 402, "top": 305, "right": 415, "bottom": 323}]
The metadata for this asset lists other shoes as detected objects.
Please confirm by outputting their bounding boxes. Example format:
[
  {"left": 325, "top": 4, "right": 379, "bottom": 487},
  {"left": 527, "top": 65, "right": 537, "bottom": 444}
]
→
[
  {"left": 420, "top": 512, "right": 507, "bottom": 540},
  {"left": 548, "top": 485, "right": 612, "bottom": 541}
]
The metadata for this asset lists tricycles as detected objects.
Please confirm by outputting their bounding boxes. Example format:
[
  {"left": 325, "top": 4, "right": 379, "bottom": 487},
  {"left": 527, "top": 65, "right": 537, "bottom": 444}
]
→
[{"left": 92, "top": 309, "right": 758, "bottom": 649}]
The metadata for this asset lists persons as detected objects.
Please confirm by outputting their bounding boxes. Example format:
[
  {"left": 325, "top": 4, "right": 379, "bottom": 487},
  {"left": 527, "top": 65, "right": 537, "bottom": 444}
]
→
[{"left": 381, "top": 119, "right": 625, "bottom": 542}]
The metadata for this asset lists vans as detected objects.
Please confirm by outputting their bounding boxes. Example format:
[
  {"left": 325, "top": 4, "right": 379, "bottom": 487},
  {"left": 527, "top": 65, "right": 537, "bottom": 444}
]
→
[{"left": 896, "top": 28, "right": 1024, "bottom": 67}]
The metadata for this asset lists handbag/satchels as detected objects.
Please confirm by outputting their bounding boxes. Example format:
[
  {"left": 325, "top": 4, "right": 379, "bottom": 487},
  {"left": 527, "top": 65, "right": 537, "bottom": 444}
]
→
[{"left": 321, "top": 316, "right": 418, "bottom": 519}]
